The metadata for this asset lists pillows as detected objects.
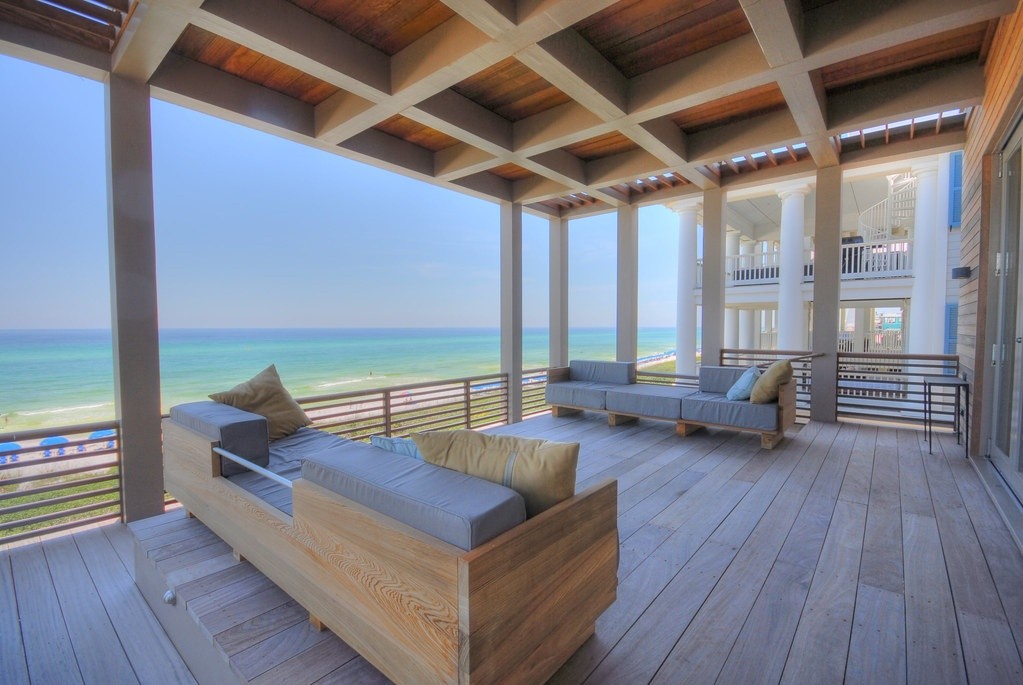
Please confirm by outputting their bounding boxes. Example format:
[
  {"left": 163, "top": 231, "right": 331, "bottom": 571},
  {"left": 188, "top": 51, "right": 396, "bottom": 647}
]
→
[
  {"left": 749, "top": 358, "right": 795, "bottom": 404},
  {"left": 207, "top": 362, "right": 315, "bottom": 441},
  {"left": 371, "top": 431, "right": 422, "bottom": 462},
  {"left": 410, "top": 429, "right": 583, "bottom": 517},
  {"left": 725, "top": 364, "right": 761, "bottom": 400}
]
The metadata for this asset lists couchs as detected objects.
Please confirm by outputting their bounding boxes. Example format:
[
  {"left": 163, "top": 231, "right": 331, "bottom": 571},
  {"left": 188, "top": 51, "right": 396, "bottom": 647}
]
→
[
  {"left": 156, "top": 397, "right": 623, "bottom": 685},
  {"left": 544, "top": 357, "right": 802, "bottom": 450}
]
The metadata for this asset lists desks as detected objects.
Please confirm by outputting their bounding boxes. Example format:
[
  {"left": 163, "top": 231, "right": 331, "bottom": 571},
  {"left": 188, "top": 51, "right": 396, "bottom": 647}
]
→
[{"left": 921, "top": 374, "right": 971, "bottom": 459}]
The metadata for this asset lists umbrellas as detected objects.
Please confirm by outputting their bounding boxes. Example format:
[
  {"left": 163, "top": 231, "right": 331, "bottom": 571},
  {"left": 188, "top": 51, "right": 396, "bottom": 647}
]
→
[
  {"left": 89, "top": 428, "right": 115, "bottom": 438},
  {"left": 0, "top": 443, "right": 21, "bottom": 452},
  {"left": 400, "top": 390, "right": 411, "bottom": 394},
  {"left": 40, "top": 437, "right": 68, "bottom": 446}
]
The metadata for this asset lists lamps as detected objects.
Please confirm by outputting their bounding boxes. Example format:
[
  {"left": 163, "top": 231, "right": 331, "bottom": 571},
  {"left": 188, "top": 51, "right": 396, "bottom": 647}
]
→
[{"left": 952, "top": 266, "right": 971, "bottom": 280}]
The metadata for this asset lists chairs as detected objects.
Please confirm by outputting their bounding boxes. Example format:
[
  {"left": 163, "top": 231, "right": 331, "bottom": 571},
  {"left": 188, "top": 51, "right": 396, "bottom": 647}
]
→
[{"left": 0, "top": 439, "right": 114, "bottom": 464}]
